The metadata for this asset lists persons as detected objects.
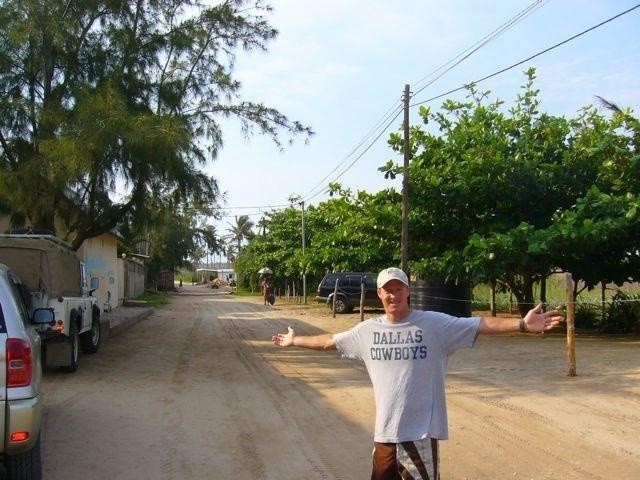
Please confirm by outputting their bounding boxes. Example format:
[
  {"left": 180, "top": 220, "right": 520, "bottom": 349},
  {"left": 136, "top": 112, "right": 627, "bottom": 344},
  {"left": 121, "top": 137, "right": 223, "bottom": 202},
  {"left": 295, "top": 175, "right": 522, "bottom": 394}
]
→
[
  {"left": 272, "top": 266, "right": 563, "bottom": 480},
  {"left": 178, "top": 278, "right": 184, "bottom": 292},
  {"left": 261, "top": 277, "right": 271, "bottom": 306}
]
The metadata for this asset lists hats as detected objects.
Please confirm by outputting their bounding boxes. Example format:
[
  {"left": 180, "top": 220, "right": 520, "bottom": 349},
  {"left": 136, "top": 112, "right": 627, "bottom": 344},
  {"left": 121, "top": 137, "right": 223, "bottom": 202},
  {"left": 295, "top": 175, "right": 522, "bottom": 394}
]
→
[{"left": 377, "top": 268, "right": 407, "bottom": 288}]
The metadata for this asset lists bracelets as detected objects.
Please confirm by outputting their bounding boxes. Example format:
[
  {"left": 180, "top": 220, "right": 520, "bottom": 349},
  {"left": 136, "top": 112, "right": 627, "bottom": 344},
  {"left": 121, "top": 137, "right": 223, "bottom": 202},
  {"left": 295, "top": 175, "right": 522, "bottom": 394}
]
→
[{"left": 519, "top": 317, "right": 525, "bottom": 332}]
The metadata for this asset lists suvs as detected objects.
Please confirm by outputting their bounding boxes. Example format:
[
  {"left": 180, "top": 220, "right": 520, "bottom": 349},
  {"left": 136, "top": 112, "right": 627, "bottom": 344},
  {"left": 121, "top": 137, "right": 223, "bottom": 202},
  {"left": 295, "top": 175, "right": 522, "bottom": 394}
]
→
[
  {"left": 315, "top": 271, "right": 385, "bottom": 315},
  {"left": 0, "top": 262, "right": 55, "bottom": 480}
]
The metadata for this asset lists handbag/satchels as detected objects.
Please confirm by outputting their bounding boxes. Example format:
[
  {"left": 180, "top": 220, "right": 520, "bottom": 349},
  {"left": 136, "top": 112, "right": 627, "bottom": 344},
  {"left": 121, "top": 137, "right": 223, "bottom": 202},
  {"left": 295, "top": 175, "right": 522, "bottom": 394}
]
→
[{"left": 269, "top": 295, "right": 275, "bottom": 305}]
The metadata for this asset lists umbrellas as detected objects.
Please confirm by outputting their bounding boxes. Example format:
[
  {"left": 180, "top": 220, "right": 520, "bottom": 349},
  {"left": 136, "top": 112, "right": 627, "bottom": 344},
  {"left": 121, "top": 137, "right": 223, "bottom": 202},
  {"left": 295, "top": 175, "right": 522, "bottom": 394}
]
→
[{"left": 257, "top": 267, "right": 273, "bottom": 275}]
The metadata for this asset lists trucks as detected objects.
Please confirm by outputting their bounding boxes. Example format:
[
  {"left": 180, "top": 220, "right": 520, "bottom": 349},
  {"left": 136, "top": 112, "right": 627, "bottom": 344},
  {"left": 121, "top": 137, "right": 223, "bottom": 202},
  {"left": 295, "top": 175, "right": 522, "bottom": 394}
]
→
[{"left": 1, "top": 231, "right": 104, "bottom": 374}]
